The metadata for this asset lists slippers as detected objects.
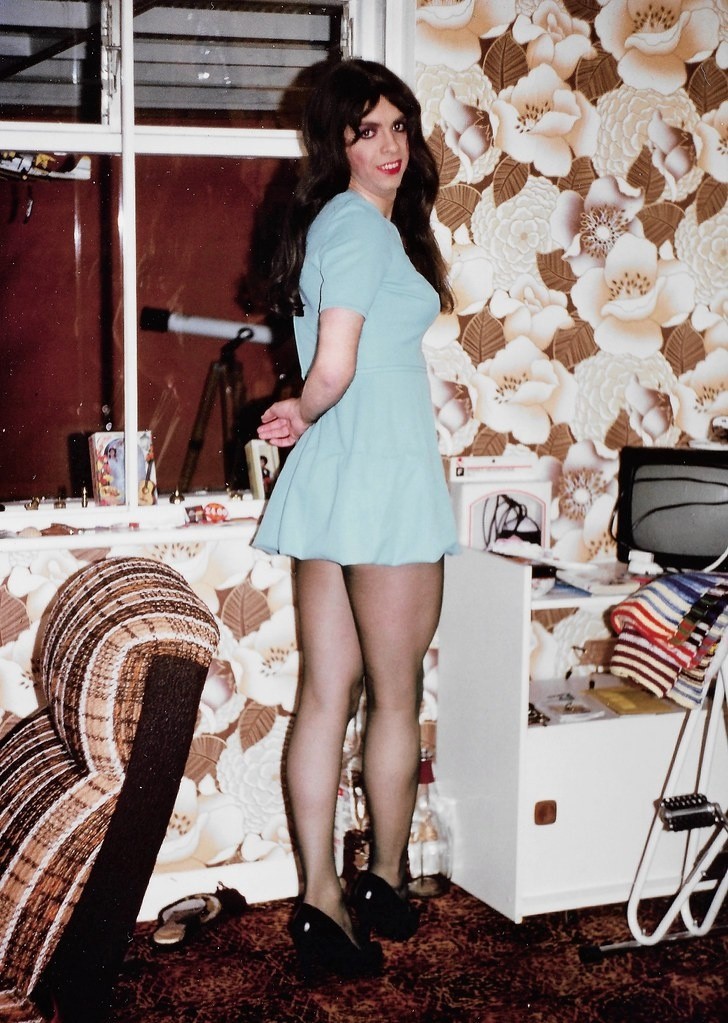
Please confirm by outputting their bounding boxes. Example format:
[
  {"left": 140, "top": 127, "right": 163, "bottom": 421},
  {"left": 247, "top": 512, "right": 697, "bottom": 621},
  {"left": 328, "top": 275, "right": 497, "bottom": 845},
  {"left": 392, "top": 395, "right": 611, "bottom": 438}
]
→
[{"left": 151, "top": 887, "right": 245, "bottom": 953}]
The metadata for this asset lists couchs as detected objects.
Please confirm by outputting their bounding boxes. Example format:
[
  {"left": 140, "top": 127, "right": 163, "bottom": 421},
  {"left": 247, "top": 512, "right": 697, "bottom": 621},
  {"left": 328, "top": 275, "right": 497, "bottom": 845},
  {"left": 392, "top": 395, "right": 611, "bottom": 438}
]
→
[{"left": 0, "top": 556, "right": 220, "bottom": 1022}]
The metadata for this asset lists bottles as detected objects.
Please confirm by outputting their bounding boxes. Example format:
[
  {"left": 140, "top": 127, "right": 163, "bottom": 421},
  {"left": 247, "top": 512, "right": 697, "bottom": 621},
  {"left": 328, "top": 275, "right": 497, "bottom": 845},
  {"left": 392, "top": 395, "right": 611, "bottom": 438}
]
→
[{"left": 403, "top": 756, "right": 454, "bottom": 897}]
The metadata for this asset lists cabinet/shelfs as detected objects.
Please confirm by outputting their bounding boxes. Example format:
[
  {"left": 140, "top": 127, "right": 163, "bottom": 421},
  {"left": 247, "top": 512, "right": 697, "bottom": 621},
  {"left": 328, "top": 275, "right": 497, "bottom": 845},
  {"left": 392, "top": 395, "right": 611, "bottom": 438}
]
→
[{"left": 440, "top": 546, "right": 727, "bottom": 924}]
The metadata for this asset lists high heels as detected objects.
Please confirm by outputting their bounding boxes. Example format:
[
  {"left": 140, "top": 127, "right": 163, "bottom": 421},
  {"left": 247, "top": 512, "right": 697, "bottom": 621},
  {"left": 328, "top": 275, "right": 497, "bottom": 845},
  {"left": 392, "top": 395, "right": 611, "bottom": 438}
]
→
[
  {"left": 287, "top": 902, "right": 382, "bottom": 986},
  {"left": 347, "top": 870, "right": 420, "bottom": 948}
]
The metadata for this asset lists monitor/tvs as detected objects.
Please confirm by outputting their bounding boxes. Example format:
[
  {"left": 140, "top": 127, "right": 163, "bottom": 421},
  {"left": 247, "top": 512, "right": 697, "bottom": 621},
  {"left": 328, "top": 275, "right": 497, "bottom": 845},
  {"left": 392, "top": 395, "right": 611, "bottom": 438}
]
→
[{"left": 617, "top": 445, "right": 728, "bottom": 574}]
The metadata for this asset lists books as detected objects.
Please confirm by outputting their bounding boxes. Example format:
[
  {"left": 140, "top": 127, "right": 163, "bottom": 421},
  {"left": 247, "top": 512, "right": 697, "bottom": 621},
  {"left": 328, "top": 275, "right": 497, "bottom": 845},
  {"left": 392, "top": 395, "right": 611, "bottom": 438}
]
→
[{"left": 557, "top": 567, "right": 638, "bottom": 596}]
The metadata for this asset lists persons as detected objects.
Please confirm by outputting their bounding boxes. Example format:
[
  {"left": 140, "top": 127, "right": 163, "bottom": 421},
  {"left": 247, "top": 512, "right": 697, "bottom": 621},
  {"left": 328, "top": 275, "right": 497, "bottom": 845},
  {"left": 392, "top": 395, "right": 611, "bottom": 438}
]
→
[
  {"left": 260, "top": 455, "right": 272, "bottom": 494},
  {"left": 107, "top": 447, "right": 125, "bottom": 496},
  {"left": 251, "top": 58, "right": 462, "bottom": 993}
]
